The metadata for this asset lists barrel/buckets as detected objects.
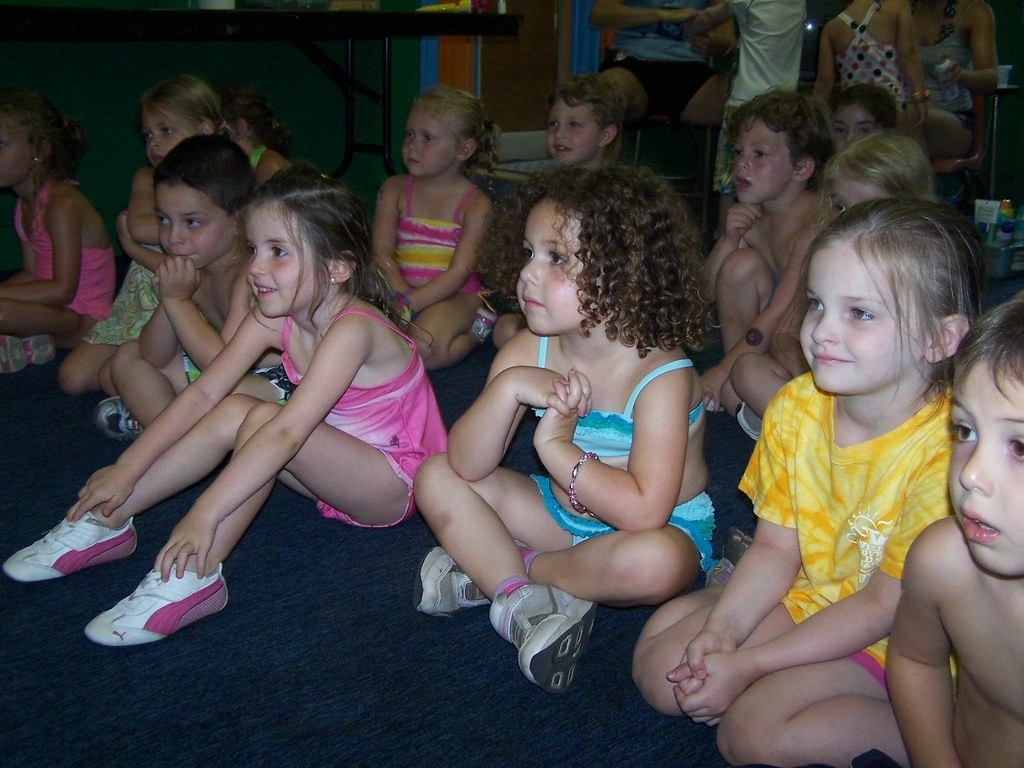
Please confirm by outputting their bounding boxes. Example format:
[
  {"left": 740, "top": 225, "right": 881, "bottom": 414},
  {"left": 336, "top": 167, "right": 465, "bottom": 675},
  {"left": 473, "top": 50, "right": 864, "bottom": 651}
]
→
[
  {"left": 943, "top": 196, "right": 968, "bottom": 219},
  {"left": 981, "top": 248, "right": 1011, "bottom": 278}
]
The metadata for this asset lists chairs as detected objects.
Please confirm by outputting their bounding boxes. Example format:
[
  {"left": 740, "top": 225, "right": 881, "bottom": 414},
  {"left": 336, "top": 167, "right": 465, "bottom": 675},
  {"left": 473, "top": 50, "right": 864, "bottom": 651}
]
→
[{"left": 930, "top": 91, "right": 984, "bottom": 208}]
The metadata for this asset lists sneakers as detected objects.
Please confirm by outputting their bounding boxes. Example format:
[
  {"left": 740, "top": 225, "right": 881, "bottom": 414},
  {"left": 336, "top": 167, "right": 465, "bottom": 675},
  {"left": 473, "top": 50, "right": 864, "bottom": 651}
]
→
[
  {"left": 470, "top": 307, "right": 498, "bottom": 343},
  {"left": 91, "top": 395, "right": 144, "bottom": 441},
  {"left": 413, "top": 540, "right": 527, "bottom": 618},
  {"left": 0, "top": 334, "right": 56, "bottom": 373},
  {"left": 2, "top": 511, "right": 138, "bottom": 582},
  {"left": 84, "top": 563, "right": 229, "bottom": 648},
  {"left": 489, "top": 582, "right": 597, "bottom": 693}
]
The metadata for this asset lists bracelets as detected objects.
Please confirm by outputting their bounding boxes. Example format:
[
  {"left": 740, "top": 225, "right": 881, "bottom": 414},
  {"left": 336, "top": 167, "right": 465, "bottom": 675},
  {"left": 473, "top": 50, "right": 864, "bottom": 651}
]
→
[
  {"left": 569, "top": 452, "right": 599, "bottom": 514},
  {"left": 911, "top": 88, "right": 929, "bottom": 104}
]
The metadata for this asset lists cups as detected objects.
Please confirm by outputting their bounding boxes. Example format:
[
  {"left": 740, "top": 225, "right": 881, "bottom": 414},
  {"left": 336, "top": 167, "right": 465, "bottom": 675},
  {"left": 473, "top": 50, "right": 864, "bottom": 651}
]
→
[{"left": 998, "top": 65, "right": 1012, "bottom": 88}]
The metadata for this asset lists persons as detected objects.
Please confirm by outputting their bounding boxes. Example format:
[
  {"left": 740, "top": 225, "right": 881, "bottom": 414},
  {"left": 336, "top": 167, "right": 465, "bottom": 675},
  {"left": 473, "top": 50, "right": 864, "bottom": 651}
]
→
[
  {"left": 833, "top": 83, "right": 897, "bottom": 147},
  {"left": 906, "top": 0, "right": 998, "bottom": 158},
  {"left": 698, "top": 89, "right": 844, "bottom": 417},
  {"left": 413, "top": 163, "right": 718, "bottom": 695},
  {"left": 588, "top": 0, "right": 738, "bottom": 126},
  {"left": 812, "top": 0, "right": 930, "bottom": 132},
  {"left": 728, "top": 134, "right": 947, "bottom": 441},
  {"left": 57, "top": 73, "right": 291, "bottom": 397},
  {"left": 369, "top": 84, "right": 498, "bottom": 371},
  {"left": 0, "top": 87, "right": 117, "bottom": 374},
  {"left": 631, "top": 197, "right": 985, "bottom": 768},
  {"left": 885, "top": 297, "right": 1024, "bottom": 768},
  {"left": 546, "top": 75, "right": 623, "bottom": 162},
  {"left": 92, "top": 135, "right": 297, "bottom": 441},
  {"left": 682, "top": 0, "right": 807, "bottom": 241},
  {"left": 2, "top": 165, "right": 448, "bottom": 647},
  {"left": 213, "top": 80, "right": 292, "bottom": 159}
]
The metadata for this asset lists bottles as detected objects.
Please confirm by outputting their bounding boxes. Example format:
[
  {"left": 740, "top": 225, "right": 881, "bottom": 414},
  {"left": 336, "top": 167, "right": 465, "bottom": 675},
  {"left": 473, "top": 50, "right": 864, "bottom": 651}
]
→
[
  {"left": 993, "top": 222, "right": 1014, "bottom": 248},
  {"left": 935, "top": 57, "right": 959, "bottom": 101},
  {"left": 997, "top": 200, "right": 1014, "bottom": 224},
  {"left": 977, "top": 221, "right": 987, "bottom": 244}
]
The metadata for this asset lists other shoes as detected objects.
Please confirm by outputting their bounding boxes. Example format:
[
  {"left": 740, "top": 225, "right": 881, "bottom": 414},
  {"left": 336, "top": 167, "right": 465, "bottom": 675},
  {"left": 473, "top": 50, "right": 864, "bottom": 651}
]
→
[
  {"left": 705, "top": 527, "right": 753, "bottom": 587},
  {"left": 735, "top": 401, "right": 762, "bottom": 441},
  {"left": 707, "top": 399, "right": 724, "bottom": 413}
]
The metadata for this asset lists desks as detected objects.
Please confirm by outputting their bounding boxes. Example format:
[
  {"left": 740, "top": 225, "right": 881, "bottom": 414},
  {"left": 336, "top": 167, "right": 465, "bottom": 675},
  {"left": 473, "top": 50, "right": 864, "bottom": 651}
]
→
[
  {"left": 986, "top": 85, "right": 1021, "bottom": 201},
  {"left": 0, "top": 6, "right": 524, "bottom": 184}
]
